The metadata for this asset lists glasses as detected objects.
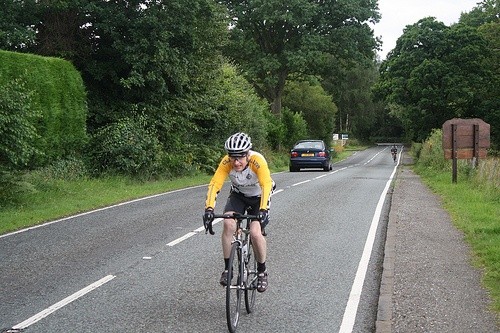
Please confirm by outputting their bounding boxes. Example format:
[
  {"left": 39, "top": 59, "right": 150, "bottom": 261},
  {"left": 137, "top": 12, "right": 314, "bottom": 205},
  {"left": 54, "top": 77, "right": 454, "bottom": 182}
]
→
[{"left": 229, "top": 156, "right": 245, "bottom": 162}]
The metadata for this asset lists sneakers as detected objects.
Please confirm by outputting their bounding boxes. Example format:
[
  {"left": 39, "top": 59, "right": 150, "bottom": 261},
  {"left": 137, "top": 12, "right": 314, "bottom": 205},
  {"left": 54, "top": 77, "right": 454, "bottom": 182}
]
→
[
  {"left": 220, "top": 270, "right": 233, "bottom": 285},
  {"left": 257, "top": 267, "right": 269, "bottom": 292}
]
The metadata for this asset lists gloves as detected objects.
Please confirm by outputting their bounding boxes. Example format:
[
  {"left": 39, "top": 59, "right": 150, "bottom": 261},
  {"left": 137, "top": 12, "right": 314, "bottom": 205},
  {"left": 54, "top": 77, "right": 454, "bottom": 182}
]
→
[
  {"left": 257, "top": 209, "right": 270, "bottom": 228},
  {"left": 203, "top": 207, "right": 214, "bottom": 225}
]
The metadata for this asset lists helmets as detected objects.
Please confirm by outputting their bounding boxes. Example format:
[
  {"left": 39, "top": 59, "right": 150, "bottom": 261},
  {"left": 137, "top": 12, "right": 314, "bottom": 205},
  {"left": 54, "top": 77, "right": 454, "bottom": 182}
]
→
[{"left": 224, "top": 133, "right": 252, "bottom": 153}]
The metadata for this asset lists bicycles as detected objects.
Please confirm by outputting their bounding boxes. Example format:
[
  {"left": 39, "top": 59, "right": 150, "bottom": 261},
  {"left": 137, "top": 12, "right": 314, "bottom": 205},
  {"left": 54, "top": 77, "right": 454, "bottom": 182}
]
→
[
  {"left": 205, "top": 211, "right": 268, "bottom": 333},
  {"left": 392, "top": 152, "right": 397, "bottom": 163}
]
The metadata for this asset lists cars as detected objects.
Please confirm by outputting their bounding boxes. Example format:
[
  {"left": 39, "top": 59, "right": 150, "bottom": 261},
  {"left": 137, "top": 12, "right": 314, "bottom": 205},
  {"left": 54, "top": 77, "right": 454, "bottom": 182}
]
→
[{"left": 289, "top": 140, "right": 333, "bottom": 172}]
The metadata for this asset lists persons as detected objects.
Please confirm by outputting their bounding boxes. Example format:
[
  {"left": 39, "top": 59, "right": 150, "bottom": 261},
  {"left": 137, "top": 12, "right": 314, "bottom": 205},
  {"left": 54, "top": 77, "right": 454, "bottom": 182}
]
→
[
  {"left": 203, "top": 132, "right": 273, "bottom": 292},
  {"left": 391, "top": 144, "right": 398, "bottom": 160}
]
